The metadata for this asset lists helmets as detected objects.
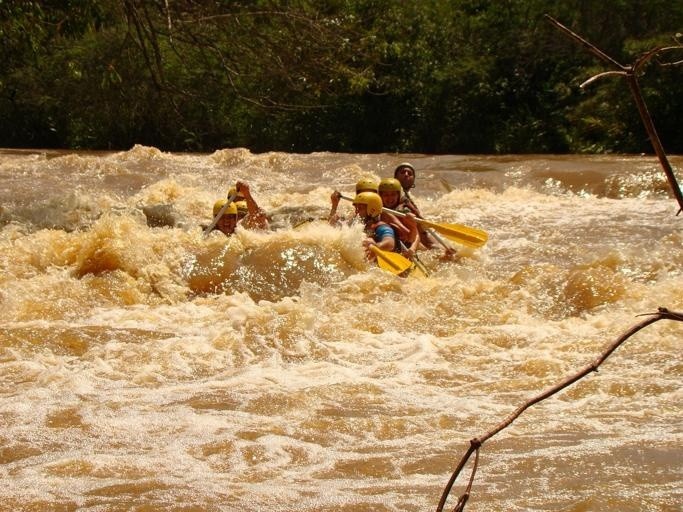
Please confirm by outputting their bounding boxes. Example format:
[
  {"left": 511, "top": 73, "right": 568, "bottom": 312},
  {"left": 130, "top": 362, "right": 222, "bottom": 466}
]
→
[
  {"left": 352, "top": 192, "right": 383, "bottom": 218},
  {"left": 234, "top": 201, "right": 248, "bottom": 215},
  {"left": 378, "top": 177, "right": 402, "bottom": 203},
  {"left": 393, "top": 162, "right": 415, "bottom": 179},
  {"left": 227, "top": 186, "right": 244, "bottom": 199},
  {"left": 354, "top": 178, "right": 378, "bottom": 194},
  {"left": 212, "top": 198, "right": 237, "bottom": 225}
]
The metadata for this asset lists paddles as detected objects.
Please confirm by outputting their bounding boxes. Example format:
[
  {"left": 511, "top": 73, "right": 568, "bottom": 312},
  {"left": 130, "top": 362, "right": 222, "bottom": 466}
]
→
[
  {"left": 333, "top": 191, "right": 488, "bottom": 248},
  {"left": 403, "top": 196, "right": 457, "bottom": 259},
  {"left": 334, "top": 219, "right": 412, "bottom": 276}
]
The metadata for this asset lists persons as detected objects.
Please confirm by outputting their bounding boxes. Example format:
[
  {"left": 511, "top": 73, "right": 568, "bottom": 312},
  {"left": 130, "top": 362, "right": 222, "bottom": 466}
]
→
[{"left": 201, "top": 161, "right": 457, "bottom": 269}]
